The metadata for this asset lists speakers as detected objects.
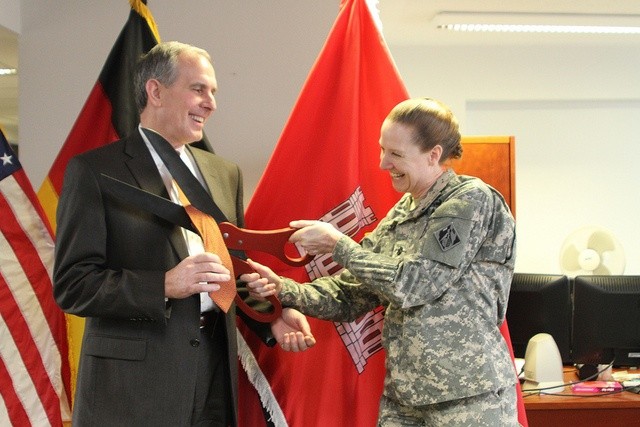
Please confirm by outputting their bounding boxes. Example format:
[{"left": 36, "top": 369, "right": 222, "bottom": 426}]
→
[{"left": 521, "top": 332, "right": 565, "bottom": 393}]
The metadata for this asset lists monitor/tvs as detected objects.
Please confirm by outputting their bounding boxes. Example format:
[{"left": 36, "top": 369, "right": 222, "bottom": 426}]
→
[
  {"left": 573, "top": 274, "right": 640, "bottom": 368},
  {"left": 506, "top": 272, "right": 570, "bottom": 365}
]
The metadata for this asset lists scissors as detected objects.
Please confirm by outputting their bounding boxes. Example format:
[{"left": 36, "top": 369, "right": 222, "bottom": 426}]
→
[{"left": 100, "top": 172, "right": 316, "bottom": 323}]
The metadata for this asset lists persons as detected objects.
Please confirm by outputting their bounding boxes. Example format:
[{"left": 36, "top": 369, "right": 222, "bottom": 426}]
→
[
  {"left": 240, "top": 95, "right": 519, "bottom": 425},
  {"left": 52, "top": 40, "right": 318, "bottom": 425}
]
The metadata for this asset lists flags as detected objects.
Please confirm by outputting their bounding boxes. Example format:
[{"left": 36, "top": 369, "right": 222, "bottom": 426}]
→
[
  {"left": 240, "top": 0, "right": 529, "bottom": 426},
  {"left": 35, "top": 0, "right": 217, "bottom": 407},
  {"left": 0, "top": 127, "right": 73, "bottom": 426}
]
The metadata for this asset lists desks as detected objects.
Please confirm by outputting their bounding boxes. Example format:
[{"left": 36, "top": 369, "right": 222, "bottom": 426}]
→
[{"left": 516, "top": 364, "right": 640, "bottom": 426}]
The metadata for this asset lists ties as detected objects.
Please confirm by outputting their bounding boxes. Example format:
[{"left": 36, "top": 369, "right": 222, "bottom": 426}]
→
[{"left": 171, "top": 177, "right": 239, "bottom": 315}]
